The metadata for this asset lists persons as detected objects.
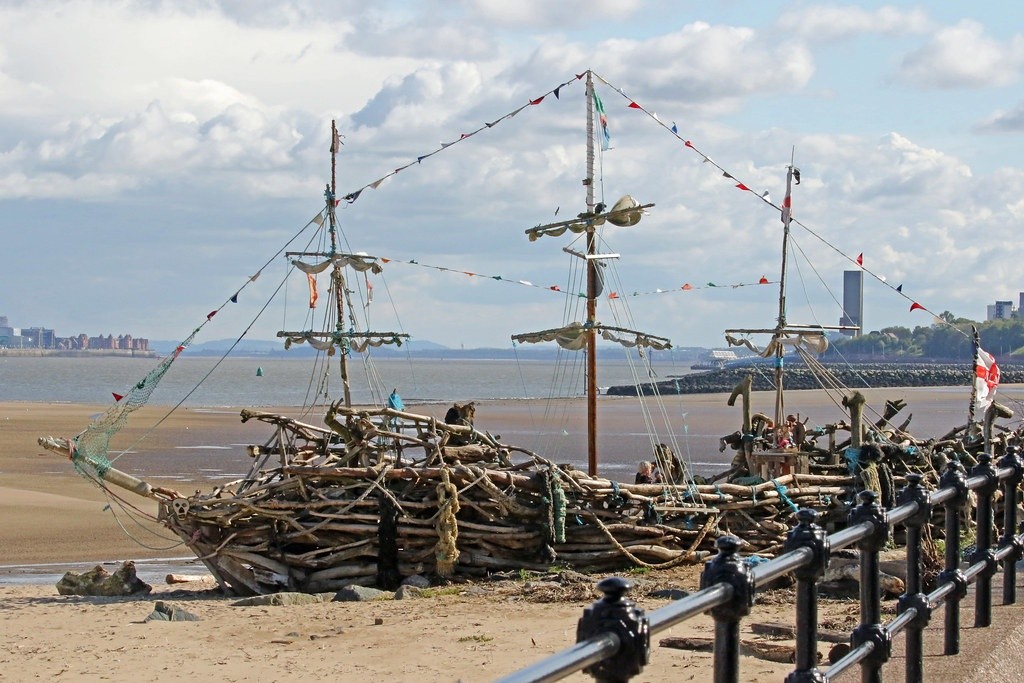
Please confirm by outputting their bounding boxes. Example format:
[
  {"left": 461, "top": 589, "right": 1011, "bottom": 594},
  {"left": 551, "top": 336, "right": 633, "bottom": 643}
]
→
[{"left": 634, "top": 459, "right": 662, "bottom": 485}]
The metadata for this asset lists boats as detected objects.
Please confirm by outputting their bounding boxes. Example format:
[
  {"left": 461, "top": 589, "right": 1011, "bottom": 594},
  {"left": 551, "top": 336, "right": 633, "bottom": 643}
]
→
[
  {"left": 632, "top": 136, "right": 1016, "bottom": 489},
  {"left": 31, "top": 64, "right": 999, "bottom": 592}
]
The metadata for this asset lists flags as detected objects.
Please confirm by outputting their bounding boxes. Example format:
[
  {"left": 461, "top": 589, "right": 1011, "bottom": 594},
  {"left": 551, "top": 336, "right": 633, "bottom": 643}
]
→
[
  {"left": 590, "top": 83, "right": 615, "bottom": 150},
  {"left": 364, "top": 274, "right": 375, "bottom": 309},
  {"left": 306, "top": 272, "right": 319, "bottom": 309},
  {"left": 976, "top": 346, "right": 1001, "bottom": 417}
]
